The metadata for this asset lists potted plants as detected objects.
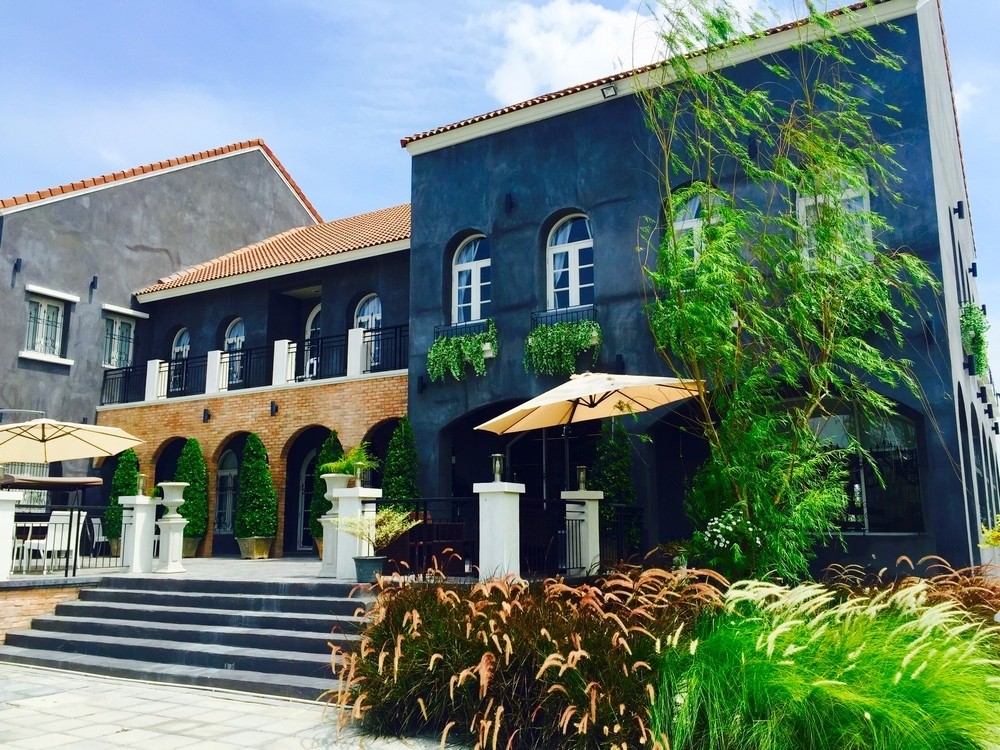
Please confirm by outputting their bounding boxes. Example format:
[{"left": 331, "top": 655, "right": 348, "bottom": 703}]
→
[
  {"left": 523, "top": 318, "right": 604, "bottom": 378},
  {"left": 427, "top": 316, "right": 500, "bottom": 384},
  {"left": 308, "top": 413, "right": 423, "bottom": 583},
  {"left": 102, "top": 437, "right": 208, "bottom": 558},
  {"left": 233, "top": 433, "right": 279, "bottom": 560}
]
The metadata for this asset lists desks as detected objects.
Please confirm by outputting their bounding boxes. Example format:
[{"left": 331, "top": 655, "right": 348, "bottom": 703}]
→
[{"left": 15, "top": 522, "right": 48, "bottom": 574}]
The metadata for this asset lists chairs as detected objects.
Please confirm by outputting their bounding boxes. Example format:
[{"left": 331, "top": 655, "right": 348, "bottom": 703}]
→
[
  {"left": 11, "top": 510, "right": 109, "bottom": 574},
  {"left": 296, "top": 357, "right": 320, "bottom": 382}
]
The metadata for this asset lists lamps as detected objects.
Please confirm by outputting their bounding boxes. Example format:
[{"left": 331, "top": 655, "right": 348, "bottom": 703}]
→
[
  {"left": 577, "top": 466, "right": 586, "bottom": 491},
  {"left": 203, "top": 409, "right": 210, "bottom": 423},
  {"left": 492, "top": 454, "right": 503, "bottom": 482},
  {"left": 91, "top": 276, "right": 98, "bottom": 289},
  {"left": 923, "top": 204, "right": 999, "bottom": 437},
  {"left": 504, "top": 192, "right": 512, "bottom": 210},
  {"left": 14, "top": 258, "right": 22, "bottom": 272},
  {"left": 354, "top": 462, "right": 364, "bottom": 487},
  {"left": 271, "top": 401, "right": 278, "bottom": 416}
]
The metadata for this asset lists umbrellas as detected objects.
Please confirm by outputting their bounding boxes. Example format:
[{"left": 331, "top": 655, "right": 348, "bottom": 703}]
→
[
  {"left": 0, "top": 419, "right": 146, "bottom": 466},
  {"left": 473, "top": 372, "right": 710, "bottom": 490}
]
